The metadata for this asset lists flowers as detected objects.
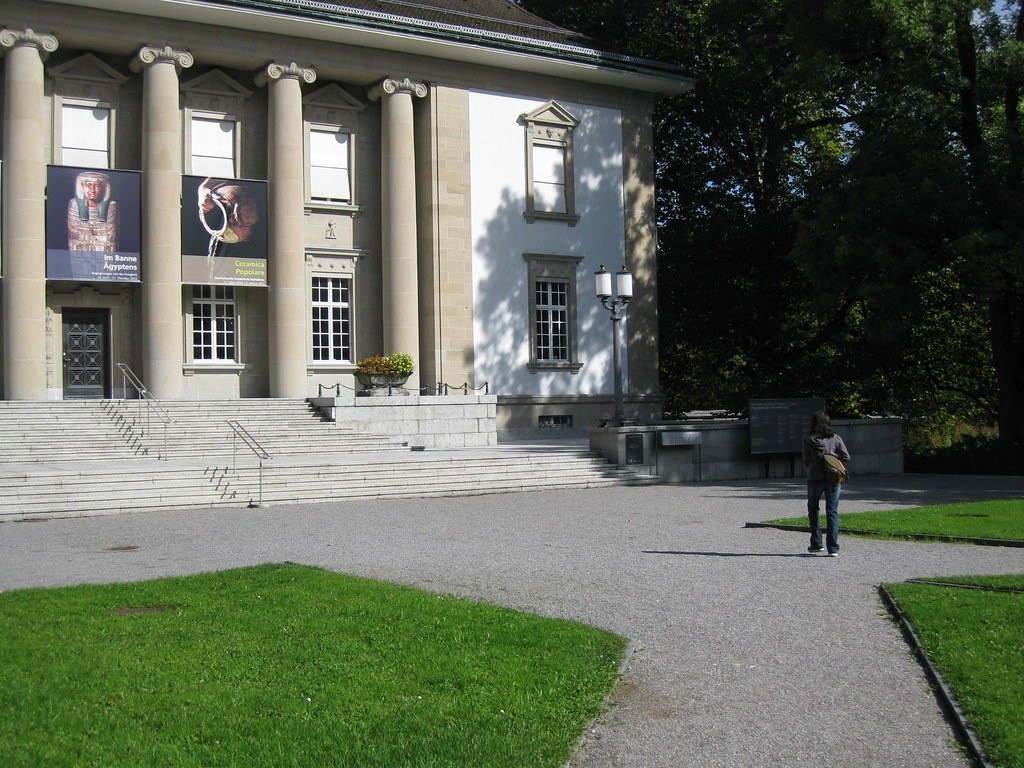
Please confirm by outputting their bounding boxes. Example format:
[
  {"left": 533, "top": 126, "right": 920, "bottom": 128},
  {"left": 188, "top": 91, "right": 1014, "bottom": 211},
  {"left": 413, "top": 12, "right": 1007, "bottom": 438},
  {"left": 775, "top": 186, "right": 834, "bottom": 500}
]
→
[{"left": 355, "top": 352, "right": 416, "bottom": 376}]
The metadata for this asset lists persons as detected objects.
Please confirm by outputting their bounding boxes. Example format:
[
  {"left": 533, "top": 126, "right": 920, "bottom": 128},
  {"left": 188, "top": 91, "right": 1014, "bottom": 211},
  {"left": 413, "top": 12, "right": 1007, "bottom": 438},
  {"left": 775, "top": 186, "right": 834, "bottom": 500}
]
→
[
  {"left": 197, "top": 177, "right": 266, "bottom": 257},
  {"left": 802, "top": 411, "right": 850, "bottom": 557}
]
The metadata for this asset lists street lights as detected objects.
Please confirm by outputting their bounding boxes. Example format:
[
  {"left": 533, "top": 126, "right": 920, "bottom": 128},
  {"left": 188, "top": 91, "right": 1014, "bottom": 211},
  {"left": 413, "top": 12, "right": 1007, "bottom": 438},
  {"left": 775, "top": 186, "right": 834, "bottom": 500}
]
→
[{"left": 592, "top": 265, "right": 633, "bottom": 429}]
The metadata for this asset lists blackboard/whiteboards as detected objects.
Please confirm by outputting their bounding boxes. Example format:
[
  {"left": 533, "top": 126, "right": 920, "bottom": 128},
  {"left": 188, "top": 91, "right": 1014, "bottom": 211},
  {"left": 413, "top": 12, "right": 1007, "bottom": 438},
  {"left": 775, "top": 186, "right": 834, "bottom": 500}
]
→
[{"left": 747, "top": 398, "right": 826, "bottom": 454}]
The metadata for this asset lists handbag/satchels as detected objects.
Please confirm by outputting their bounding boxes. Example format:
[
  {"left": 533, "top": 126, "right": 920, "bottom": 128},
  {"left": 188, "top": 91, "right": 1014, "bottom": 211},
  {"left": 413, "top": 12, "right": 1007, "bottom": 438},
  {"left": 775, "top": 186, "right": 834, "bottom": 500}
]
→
[{"left": 821, "top": 454, "right": 849, "bottom": 483}]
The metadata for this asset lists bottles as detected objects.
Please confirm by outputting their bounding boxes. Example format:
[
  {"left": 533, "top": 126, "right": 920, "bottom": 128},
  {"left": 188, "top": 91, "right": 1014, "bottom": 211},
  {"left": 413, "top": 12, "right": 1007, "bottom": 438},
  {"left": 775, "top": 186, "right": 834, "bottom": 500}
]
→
[{"left": 198, "top": 187, "right": 260, "bottom": 244}]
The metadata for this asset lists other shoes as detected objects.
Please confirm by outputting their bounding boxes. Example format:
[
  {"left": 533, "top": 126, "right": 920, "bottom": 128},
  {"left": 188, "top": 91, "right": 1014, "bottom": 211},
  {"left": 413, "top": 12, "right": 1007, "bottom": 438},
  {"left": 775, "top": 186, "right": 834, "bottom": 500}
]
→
[
  {"left": 828, "top": 551, "right": 838, "bottom": 557},
  {"left": 808, "top": 546, "right": 824, "bottom": 552}
]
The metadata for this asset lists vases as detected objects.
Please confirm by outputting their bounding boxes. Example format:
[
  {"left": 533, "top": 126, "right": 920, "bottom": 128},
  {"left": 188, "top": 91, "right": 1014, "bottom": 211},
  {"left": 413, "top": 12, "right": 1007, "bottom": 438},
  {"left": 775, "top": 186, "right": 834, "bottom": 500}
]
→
[{"left": 355, "top": 372, "right": 413, "bottom": 388}]
